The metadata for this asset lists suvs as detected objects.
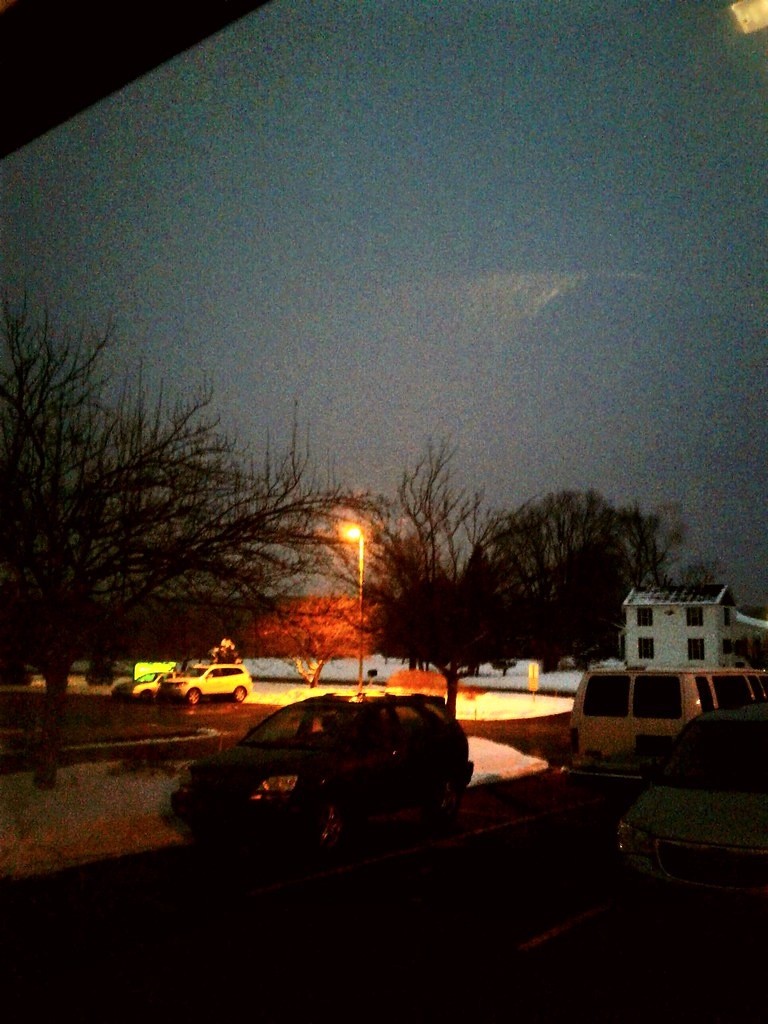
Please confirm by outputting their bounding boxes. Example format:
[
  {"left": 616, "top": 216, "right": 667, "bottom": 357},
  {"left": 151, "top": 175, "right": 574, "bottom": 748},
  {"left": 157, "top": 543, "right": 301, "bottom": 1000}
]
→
[{"left": 164, "top": 664, "right": 252, "bottom": 705}]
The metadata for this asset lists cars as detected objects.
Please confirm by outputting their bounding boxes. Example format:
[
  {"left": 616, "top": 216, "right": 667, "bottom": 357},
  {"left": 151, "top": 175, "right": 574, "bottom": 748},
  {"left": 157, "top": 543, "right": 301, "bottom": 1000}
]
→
[
  {"left": 111, "top": 672, "right": 167, "bottom": 700},
  {"left": 168, "top": 689, "right": 472, "bottom": 854},
  {"left": 619, "top": 701, "right": 768, "bottom": 902}
]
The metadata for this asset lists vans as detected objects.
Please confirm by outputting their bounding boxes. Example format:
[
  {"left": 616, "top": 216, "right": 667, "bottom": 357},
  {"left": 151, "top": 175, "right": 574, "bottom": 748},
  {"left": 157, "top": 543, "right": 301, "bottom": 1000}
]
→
[{"left": 571, "top": 665, "right": 766, "bottom": 794}]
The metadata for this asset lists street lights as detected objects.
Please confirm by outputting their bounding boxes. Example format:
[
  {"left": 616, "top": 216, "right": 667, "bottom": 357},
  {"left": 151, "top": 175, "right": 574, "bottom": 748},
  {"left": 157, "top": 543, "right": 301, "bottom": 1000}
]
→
[{"left": 342, "top": 524, "right": 364, "bottom": 692}]
